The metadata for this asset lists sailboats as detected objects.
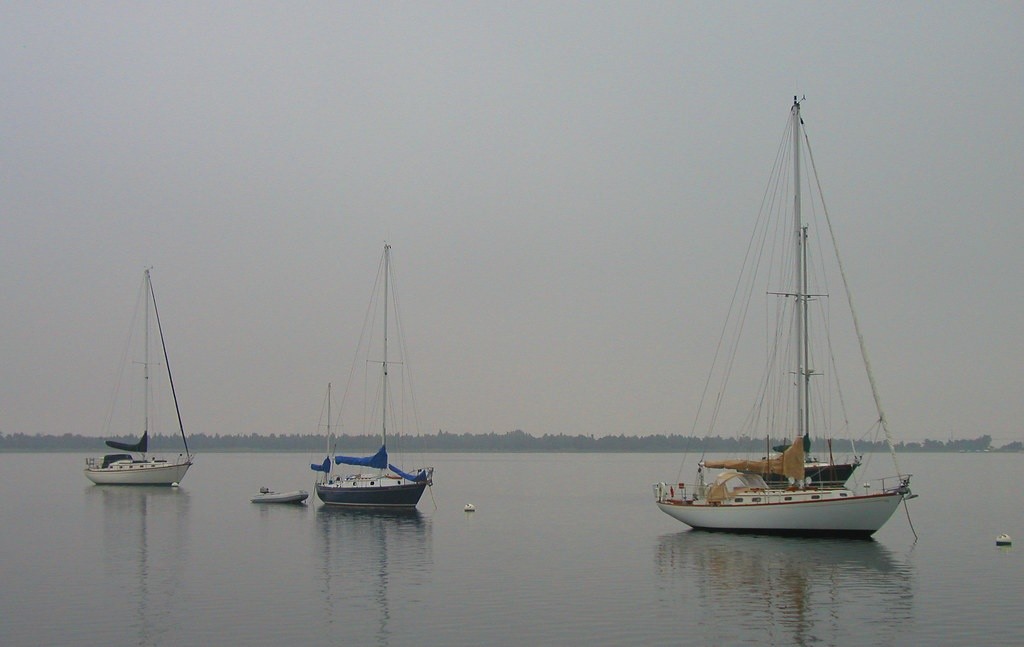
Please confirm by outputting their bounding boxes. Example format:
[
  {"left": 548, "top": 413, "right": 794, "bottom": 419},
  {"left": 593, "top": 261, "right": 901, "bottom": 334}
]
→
[
  {"left": 315, "top": 245, "right": 439, "bottom": 513},
  {"left": 656, "top": 93, "right": 924, "bottom": 539},
  {"left": 84, "top": 263, "right": 194, "bottom": 488}
]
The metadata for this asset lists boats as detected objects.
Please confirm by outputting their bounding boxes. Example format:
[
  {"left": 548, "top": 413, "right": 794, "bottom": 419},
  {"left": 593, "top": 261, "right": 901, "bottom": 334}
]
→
[{"left": 248, "top": 486, "right": 309, "bottom": 503}]
